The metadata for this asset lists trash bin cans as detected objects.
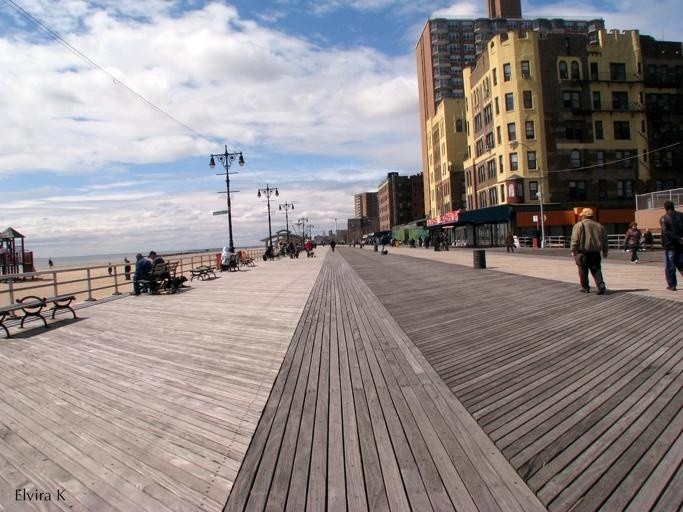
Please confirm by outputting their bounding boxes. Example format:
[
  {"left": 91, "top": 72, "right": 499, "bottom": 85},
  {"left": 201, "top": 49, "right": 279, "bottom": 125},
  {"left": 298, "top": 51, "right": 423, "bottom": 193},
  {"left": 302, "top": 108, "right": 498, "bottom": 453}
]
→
[
  {"left": 374, "top": 244, "right": 378, "bottom": 251},
  {"left": 433, "top": 238, "right": 440, "bottom": 251},
  {"left": 473, "top": 250, "right": 486, "bottom": 268},
  {"left": 533, "top": 236, "right": 539, "bottom": 248}
]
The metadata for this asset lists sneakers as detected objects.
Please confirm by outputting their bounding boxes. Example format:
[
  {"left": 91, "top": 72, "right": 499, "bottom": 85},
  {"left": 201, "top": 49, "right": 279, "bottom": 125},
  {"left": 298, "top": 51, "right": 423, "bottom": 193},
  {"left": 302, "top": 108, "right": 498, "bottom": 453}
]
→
[
  {"left": 579, "top": 287, "right": 591, "bottom": 293},
  {"left": 595, "top": 286, "right": 605, "bottom": 295},
  {"left": 629, "top": 259, "right": 638, "bottom": 265},
  {"left": 666, "top": 286, "right": 676, "bottom": 291}
]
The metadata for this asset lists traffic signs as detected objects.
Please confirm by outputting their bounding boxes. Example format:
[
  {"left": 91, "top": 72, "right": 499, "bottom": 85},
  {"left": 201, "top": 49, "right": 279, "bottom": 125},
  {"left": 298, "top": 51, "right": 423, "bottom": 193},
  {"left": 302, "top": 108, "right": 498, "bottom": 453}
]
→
[{"left": 211, "top": 210, "right": 227, "bottom": 216}]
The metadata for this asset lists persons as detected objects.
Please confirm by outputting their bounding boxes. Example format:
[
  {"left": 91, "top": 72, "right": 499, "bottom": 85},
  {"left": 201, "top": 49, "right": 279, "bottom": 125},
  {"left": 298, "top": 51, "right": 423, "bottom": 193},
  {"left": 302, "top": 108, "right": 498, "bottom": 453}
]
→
[
  {"left": 149, "top": 251, "right": 168, "bottom": 295},
  {"left": 130, "top": 253, "right": 152, "bottom": 296},
  {"left": 48, "top": 259, "right": 53, "bottom": 267},
  {"left": 124, "top": 257, "right": 131, "bottom": 280},
  {"left": 659, "top": 201, "right": 683, "bottom": 291},
  {"left": 643, "top": 228, "right": 654, "bottom": 251},
  {"left": 622, "top": 221, "right": 642, "bottom": 263},
  {"left": 570, "top": 207, "right": 608, "bottom": 295},
  {"left": 506, "top": 232, "right": 516, "bottom": 252},
  {"left": 108, "top": 262, "right": 112, "bottom": 275},
  {"left": 409, "top": 231, "right": 449, "bottom": 251},
  {"left": 220, "top": 234, "right": 397, "bottom": 271}
]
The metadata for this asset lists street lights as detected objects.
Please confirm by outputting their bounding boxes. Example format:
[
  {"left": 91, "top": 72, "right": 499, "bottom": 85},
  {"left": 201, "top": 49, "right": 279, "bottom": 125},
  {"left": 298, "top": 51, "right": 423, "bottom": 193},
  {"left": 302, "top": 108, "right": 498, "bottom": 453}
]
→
[
  {"left": 508, "top": 139, "right": 546, "bottom": 250},
  {"left": 278, "top": 199, "right": 315, "bottom": 248},
  {"left": 207, "top": 144, "right": 246, "bottom": 254},
  {"left": 256, "top": 183, "right": 280, "bottom": 246}
]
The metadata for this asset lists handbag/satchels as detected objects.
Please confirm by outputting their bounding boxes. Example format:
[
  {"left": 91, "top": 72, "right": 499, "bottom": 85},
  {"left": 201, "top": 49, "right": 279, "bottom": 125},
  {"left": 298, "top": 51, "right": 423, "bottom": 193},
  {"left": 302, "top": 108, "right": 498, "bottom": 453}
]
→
[{"left": 575, "top": 254, "right": 587, "bottom": 267}]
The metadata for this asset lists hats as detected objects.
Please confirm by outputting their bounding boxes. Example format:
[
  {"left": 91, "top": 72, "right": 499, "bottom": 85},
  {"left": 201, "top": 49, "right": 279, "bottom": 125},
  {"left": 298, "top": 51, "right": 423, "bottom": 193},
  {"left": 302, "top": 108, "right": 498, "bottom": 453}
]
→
[{"left": 578, "top": 207, "right": 594, "bottom": 217}]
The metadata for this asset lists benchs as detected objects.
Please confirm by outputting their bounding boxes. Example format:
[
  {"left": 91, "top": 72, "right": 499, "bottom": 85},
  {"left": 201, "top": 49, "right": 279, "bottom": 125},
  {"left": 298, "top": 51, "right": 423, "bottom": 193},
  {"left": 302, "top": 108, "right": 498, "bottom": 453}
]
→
[
  {"left": 190, "top": 265, "right": 216, "bottom": 282},
  {"left": 0, "top": 294, "right": 76, "bottom": 338},
  {"left": 238, "top": 251, "right": 254, "bottom": 267},
  {"left": 137, "top": 260, "right": 179, "bottom": 295},
  {"left": 639, "top": 232, "right": 663, "bottom": 251},
  {"left": 607, "top": 234, "right": 627, "bottom": 249},
  {"left": 514, "top": 237, "right": 531, "bottom": 247},
  {"left": 544, "top": 236, "right": 566, "bottom": 248},
  {"left": 223, "top": 255, "right": 239, "bottom": 272}
]
